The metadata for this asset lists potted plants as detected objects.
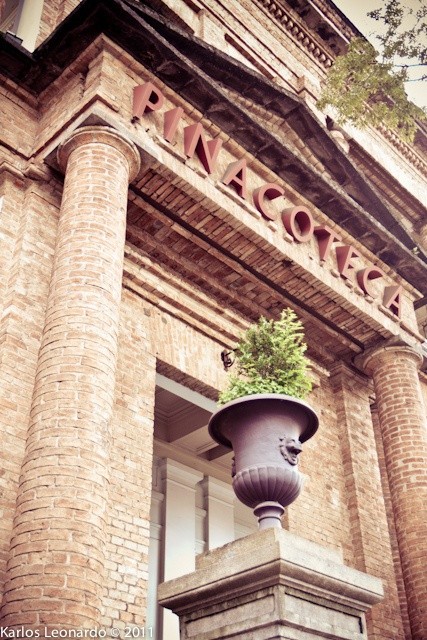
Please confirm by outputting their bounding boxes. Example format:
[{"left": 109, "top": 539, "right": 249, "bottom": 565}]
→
[{"left": 208, "top": 304, "right": 320, "bottom": 530}]
